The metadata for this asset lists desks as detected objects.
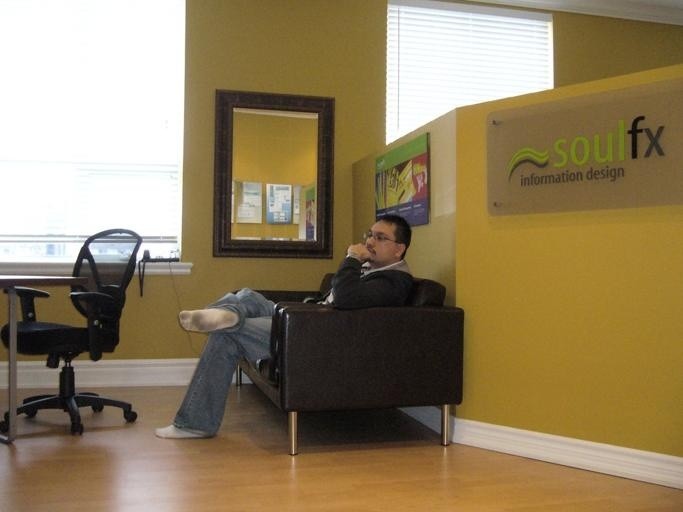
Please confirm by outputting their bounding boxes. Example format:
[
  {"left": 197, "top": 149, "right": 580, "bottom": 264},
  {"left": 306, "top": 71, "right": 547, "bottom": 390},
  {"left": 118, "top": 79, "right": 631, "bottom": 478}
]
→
[{"left": 0, "top": 273, "right": 88, "bottom": 445}]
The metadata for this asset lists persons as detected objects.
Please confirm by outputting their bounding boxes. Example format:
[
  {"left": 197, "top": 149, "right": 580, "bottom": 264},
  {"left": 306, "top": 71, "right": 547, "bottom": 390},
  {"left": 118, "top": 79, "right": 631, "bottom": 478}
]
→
[{"left": 155, "top": 215, "right": 413, "bottom": 438}]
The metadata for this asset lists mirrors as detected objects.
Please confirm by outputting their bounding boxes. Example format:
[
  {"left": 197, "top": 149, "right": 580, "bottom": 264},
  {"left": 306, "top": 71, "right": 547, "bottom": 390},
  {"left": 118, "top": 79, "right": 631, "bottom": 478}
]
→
[{"left": 213, "top": 87, "right": 334, "bottom": 260}]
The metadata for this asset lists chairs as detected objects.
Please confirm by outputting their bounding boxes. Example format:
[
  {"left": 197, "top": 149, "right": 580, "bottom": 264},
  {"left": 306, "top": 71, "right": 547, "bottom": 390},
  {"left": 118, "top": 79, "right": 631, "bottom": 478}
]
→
[
  {"left": 3, "top": 230, "right": 141, "bottom": 439},
  {"left": 232, "top": 273, "right": 463, "bottom": 457}
]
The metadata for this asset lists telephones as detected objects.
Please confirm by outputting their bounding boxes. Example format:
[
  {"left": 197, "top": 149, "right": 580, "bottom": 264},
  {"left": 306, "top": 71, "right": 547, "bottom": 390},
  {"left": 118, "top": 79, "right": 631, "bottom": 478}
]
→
[{"left": 143, "top": 250, "right": 179, "bottom": 262}]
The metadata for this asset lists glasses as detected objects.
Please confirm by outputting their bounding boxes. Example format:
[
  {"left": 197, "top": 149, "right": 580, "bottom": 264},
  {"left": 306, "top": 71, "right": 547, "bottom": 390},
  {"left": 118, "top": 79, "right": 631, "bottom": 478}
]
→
[{"left": 364, "top": 232, "right": 396, "bottom": 242}]
